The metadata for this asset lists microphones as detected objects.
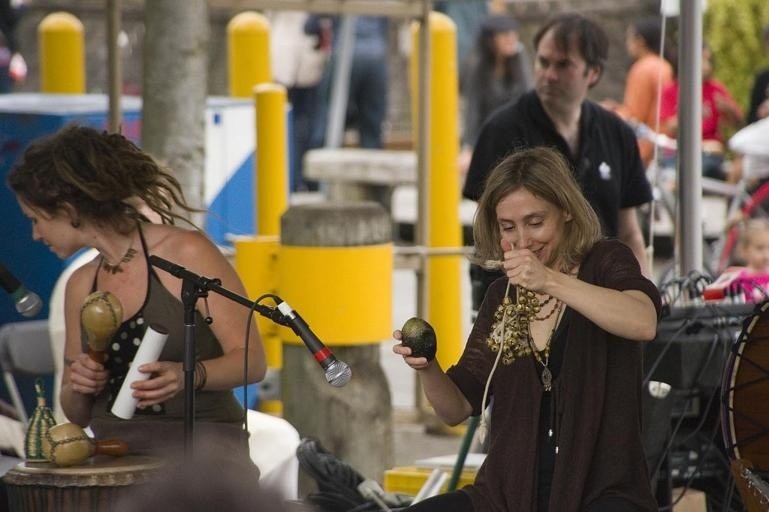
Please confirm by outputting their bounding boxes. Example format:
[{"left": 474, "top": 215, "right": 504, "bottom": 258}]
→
[
  {"left": 0, "top": 264, "right": 43, "bottom": 318},
  {"left": 274, "top": 296, "right": 352, "bottom": 389}
]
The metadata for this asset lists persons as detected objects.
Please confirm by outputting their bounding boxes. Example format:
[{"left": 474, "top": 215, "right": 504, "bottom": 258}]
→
[
  {"left": 388, "top": 144, "right": 666, "bottom": 511},
  {"left": 461, "top": 14, "right": 769, "bottom": 306},
  {"left": 11, "top": 119, "right": 268, "bottom": 485},
  {"left": 263, "top": 0, "right": 533, "bottom": 194}
]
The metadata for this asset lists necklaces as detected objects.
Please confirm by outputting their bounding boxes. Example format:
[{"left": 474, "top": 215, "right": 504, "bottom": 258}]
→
[
  {"left": 97, "top": 230, "right": 140, "bottom": 274},
  {"left": 522, "top": 287, "right": 567, "bottom": 393}
]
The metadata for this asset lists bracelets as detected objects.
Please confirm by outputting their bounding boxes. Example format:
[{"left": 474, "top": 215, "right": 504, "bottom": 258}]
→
[{"left": 193, "top": 359, "right": 207, "bottom": 393}]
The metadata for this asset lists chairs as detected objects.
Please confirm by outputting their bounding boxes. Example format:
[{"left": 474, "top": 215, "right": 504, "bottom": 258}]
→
[
  {"left": 49, "top": 248, "right": 304, "bottom": 504},
  {"left": 642, "top": 381, "right": 675, "bottom": 511}
]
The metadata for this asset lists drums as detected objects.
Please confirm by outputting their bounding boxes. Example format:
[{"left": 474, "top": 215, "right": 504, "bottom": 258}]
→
[{"left": 720, "top": 297, "right": 769, "bottom": 511}]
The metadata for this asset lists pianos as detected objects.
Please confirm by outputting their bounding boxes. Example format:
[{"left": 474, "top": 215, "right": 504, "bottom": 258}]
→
[{"left": 657, "top": 302, "right": 758, "bottom": 334}]
